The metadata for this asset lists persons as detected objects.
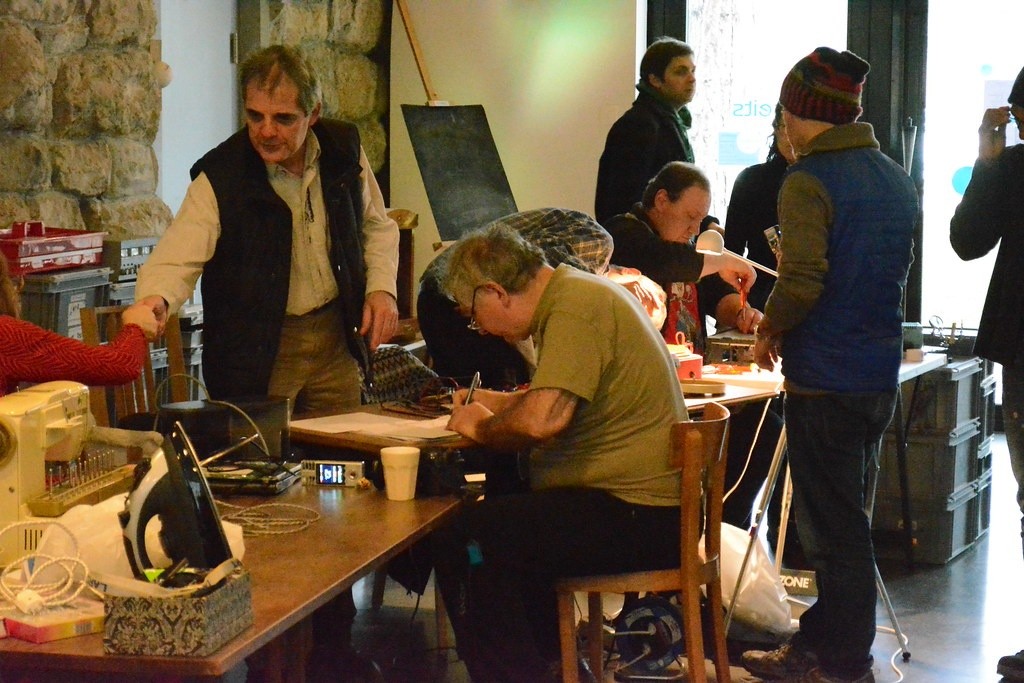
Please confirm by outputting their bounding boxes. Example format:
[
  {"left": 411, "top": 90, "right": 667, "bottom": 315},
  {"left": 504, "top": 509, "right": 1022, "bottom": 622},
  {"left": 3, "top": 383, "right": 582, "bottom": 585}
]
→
[
  {"left": 724, "top": 98, "right": 797, "bottom": 552},
  {"left": 0, "top": 248, "right": 160, "bottom": 389},
  {"left": 737, "top": 45, "right": 924, "bottom": 681},
  {"left": 611, "top": 160, "right": 765, "bottom": 338},
  {"left": 946, "top": 59, "right": 1024, "bottom": 682},
  {"left": 593, "top": 35, "right": 726, "bottom": 246},
  {"left": 124, "top": 44, "right": 404, "bottom": 683},
  {"left": 432, "top": 220, "right": 709, "bottom": 682},
  {"left": 415, "top": 206, "right": 670, "bottom": 395}
]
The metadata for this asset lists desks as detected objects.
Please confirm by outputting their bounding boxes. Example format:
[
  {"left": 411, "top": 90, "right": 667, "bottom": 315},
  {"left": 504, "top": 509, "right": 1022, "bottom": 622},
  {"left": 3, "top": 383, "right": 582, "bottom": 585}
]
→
[
  {"left": 287, "top": 402, "right": 480, "bottom": 665},
  {"left": 682, "top": 350, "right": 948, "bottom": 571},
  {"left": 0, "top": 485, "right": 465, "bottom": 683}
]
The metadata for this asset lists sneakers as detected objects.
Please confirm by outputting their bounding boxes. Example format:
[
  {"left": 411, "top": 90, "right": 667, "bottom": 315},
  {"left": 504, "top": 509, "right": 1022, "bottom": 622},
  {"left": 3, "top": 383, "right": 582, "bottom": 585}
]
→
[
  {"left": 781, "top": 665, "right": 876, "bottom": 683},
  {"left": 739, "top": 641, "right": 815, "bottom": 679}
]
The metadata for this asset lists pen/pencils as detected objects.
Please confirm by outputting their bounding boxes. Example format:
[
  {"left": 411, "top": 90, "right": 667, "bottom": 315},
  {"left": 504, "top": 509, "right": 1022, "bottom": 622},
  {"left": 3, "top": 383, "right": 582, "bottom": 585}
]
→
[{"left": 464, "top": 372, "right": 480, "bottom": 405}]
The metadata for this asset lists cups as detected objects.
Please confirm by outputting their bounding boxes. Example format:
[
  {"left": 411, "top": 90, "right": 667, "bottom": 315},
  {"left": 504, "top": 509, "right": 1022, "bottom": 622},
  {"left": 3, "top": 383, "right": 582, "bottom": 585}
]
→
[{"left": 380, "top": 448, "right": 420, "bottom": 503}]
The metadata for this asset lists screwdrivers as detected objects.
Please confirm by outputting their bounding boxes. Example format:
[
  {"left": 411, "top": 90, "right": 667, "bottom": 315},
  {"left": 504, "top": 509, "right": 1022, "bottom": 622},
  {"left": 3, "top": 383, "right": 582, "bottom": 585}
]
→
[{"left": 740, "top": 278, "right": 746, "bottom": 321}]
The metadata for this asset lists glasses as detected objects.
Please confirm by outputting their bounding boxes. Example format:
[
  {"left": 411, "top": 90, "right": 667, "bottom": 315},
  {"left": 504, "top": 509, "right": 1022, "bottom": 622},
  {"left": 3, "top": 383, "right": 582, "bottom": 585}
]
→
[
  {"left": 467, "top": 285, "right": 485, "bottom": 331},
  {"left": 1008, "top": 108, "right": 1024, "bottom": 128}
]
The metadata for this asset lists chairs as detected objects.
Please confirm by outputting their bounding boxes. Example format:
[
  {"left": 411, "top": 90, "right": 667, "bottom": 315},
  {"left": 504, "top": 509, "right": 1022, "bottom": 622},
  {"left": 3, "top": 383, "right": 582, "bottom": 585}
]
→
[
  {"left": 79, "top": 302, "right": 190, "bottom": 428},
  {"left": 554, "top": 400, "right": 732, "bottom": 683}
]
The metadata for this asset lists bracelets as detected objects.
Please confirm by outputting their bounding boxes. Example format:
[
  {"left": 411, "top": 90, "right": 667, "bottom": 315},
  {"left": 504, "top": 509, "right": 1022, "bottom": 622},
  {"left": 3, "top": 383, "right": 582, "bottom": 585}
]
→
[{"left": 753, "top": 323, "right": 771, "bottom": 340}]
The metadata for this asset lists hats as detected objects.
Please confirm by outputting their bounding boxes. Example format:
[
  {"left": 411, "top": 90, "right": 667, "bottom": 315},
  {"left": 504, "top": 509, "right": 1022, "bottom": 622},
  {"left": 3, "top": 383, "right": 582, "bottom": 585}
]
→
[
  {"left": 778, "top": 47, "right": 872, "bottom": 124},
  {"left": 1007, "top": 67, "right": 1024, "bottom": 108}
]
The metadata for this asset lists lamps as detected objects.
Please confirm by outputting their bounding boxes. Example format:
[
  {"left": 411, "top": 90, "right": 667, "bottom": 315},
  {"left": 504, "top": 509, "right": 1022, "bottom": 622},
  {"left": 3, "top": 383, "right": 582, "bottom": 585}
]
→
[{"left": 694, "top": 229, "right": 779, "bottom": 290}]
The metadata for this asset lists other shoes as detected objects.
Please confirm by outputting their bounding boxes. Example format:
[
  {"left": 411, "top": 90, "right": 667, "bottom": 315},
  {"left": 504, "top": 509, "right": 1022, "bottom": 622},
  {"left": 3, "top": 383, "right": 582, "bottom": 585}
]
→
[{"left": 997, "top": 649, "right": 1024, "bottom": 681}]
